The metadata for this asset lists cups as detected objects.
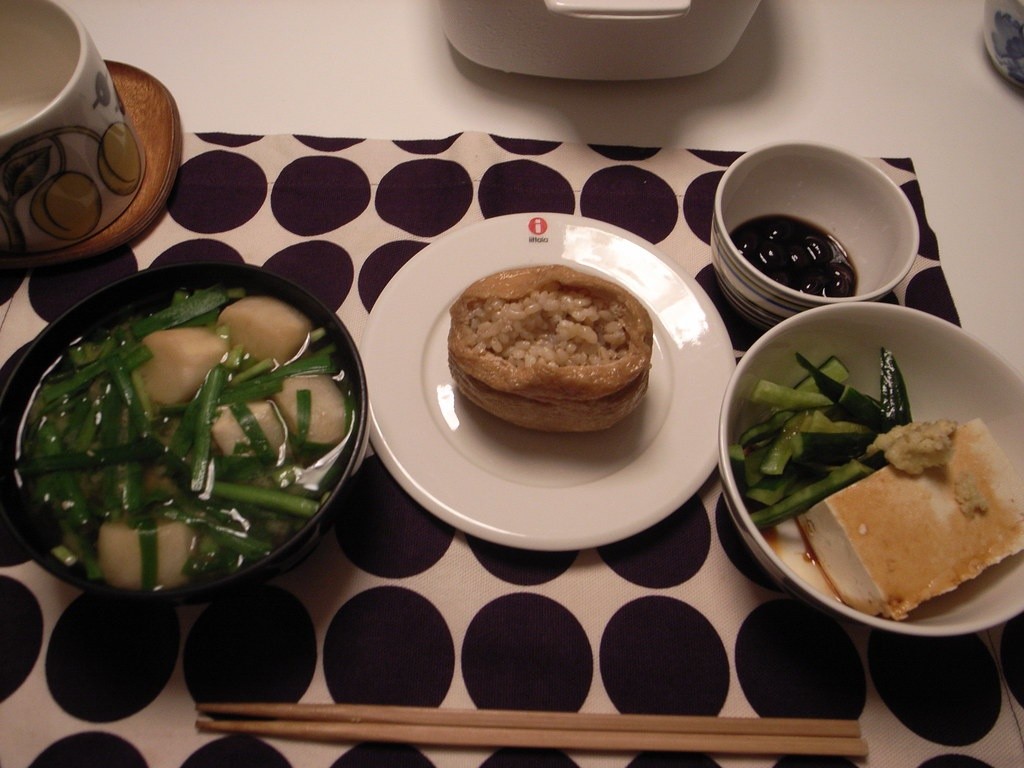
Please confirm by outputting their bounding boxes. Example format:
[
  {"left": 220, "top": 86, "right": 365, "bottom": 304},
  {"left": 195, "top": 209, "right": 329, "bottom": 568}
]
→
[
  {"left": 985, "top": 0, "right": 1024, "bottom": 87},
  {"left": 1, "top": 0, "right": 146, "bottom": 258}
]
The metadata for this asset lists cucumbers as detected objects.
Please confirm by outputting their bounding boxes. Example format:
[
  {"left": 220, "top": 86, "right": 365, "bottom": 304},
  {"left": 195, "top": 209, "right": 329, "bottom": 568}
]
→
[{"left": 725, "top": 344, "right": 915, "bottom": 531}]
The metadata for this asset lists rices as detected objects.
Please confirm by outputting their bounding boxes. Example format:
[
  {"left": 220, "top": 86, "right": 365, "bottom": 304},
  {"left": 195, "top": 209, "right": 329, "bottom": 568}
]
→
[{"left": 459, "top": 282, "right": 630, "bottom": 369}]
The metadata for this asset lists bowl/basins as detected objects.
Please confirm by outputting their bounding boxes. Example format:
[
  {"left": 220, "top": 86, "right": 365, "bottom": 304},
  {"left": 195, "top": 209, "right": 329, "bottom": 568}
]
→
[
  {"left": 718, "top": 302, "right": 1024, "bottom": 637},
  {"left": 436, "top": 0, "right": 762, "bottom": 84},
  {"left": 1, "top": 261, "right": 368, "bottom": 606},
  {"left": 710, "top": 142, "right": 921, "bottom": 329}
]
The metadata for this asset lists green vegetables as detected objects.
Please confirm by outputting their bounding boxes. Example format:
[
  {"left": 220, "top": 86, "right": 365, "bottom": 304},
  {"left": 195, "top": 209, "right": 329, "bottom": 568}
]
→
[{"left": 20, "top": 284, "right": 361, "bottom": 589}]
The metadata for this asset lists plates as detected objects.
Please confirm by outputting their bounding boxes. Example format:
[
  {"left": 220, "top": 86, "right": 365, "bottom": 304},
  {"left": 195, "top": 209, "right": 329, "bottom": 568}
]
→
[{"left": 364, "top": 213, "right": 736, "bottom": 551}]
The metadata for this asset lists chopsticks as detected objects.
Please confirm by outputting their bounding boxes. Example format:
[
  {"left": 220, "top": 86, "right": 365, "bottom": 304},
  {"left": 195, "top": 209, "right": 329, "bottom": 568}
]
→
[{"left": 195, "top": 702, "right": 870, "bottom": 759}]
